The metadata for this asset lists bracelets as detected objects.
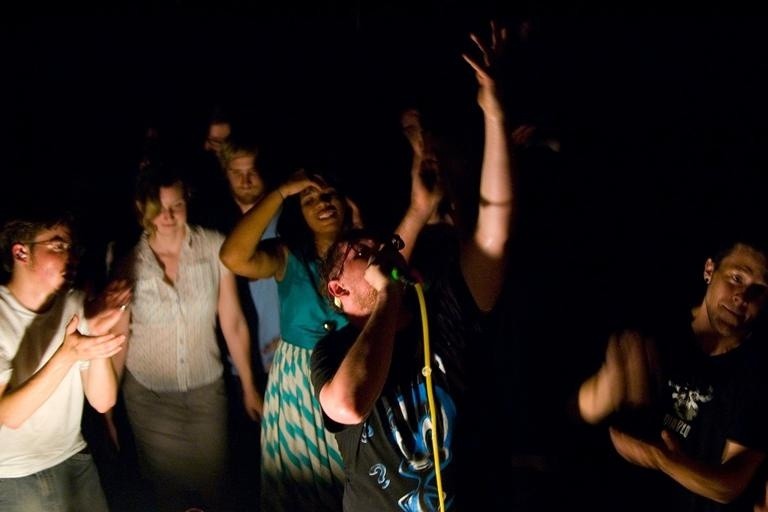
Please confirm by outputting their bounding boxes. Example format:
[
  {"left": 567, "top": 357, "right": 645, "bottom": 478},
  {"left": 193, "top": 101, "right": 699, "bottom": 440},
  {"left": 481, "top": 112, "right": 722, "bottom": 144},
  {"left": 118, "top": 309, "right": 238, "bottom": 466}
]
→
[{"left": 275, "top": 188, "right": 287, "bottom": 205}]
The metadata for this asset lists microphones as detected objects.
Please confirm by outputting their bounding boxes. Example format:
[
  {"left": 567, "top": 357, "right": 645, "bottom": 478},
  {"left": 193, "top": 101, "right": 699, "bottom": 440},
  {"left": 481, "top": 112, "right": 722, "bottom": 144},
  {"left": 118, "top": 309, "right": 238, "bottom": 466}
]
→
[{"left": 385, "top": 262, "right": 417, "bottom": 289}]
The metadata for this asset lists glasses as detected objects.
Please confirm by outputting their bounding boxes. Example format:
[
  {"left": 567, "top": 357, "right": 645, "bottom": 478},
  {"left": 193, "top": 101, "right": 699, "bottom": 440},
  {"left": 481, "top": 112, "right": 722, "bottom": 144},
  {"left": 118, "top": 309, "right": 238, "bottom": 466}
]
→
[{"left": 336, "top": 233, "right": 405, "bottom": 278}]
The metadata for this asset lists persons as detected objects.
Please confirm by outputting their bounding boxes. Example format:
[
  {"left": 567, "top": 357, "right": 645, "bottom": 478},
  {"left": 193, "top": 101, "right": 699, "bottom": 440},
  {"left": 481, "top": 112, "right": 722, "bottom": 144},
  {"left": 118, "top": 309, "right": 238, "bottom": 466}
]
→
[
  {"left": 218, "top": 99, "right": 448, "bottom": 512},
  {"left": 184, "top": 116, "right": 243, "bottom": 234},
  {"left": 309, "top": 14, "right": 532, "bottom": 512},
  {"left": 575, "top": 233, "right": 765, "bottom": 510},
  {"left": 1, "top": 198, "right": 129, "bottom": 511},
  {"left": 100, "top": 162, "right": 265, "bottom": 509},
  {"left": 207, "top": 142, "right": 292, "bottom": 512}
]
